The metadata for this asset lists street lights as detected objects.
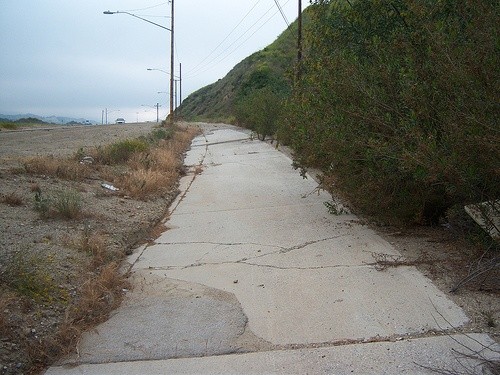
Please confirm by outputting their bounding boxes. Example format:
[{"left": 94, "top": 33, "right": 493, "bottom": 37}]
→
[
  {"left": 103, "top": 10, "right": 175, "bottom": 127},
  {"left": 147, "top": 68, "right": 181, "bottom": 106}
]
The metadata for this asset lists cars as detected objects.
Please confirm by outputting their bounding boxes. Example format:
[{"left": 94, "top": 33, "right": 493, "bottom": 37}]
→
[
  {"left": 115, "top": 118, "right": 126, "bottom": 124},
  {"left": 85, "top": 120, "right": 92, "bottom": 125}
]
